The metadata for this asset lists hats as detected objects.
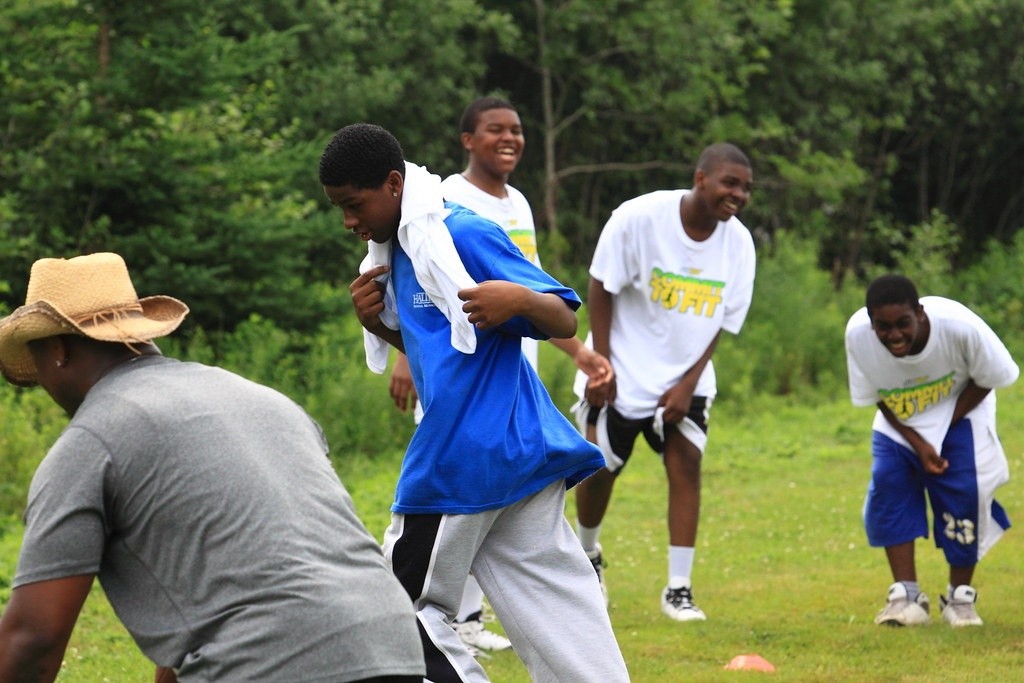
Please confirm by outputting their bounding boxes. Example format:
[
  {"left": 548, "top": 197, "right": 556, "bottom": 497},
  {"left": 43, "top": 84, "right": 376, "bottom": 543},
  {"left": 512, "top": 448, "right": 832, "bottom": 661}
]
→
[{"left": 1, "top": 252, "right": 191, "bottom": 385}]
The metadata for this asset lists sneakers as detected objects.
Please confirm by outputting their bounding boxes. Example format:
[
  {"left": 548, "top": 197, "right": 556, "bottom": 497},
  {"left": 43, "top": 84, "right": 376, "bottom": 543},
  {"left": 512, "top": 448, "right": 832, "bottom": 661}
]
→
[
  {"left": 874, "top": 581, "right": 930, "bottom": 627},
  {"left": 662, "top": 576, "right": 707, "bottom": 622},
  {"left": 452, "top": 619, "right": 512, "bottom": 651},
  {"left": 940, "top": 585, "right": 986, "bottom": 628},
  {"left": 585, "top": 542, "right": 609, "bottom": 607}
]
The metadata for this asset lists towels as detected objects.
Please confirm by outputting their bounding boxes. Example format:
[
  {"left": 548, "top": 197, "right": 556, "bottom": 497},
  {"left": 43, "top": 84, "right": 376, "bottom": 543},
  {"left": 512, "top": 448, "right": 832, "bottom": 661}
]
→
[{"left": 355, "top": 159, "right": 483, "bottom": 374}]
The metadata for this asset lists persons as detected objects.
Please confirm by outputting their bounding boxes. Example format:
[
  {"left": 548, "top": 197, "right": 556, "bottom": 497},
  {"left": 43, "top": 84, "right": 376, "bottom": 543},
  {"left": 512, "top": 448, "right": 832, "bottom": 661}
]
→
[
  {"left": 318, "top": 124, "right": 632, "bottom": 683},
  {"left": 573, "top": 142, "right": 759, "bottom": 623},
  {"left": 844, "top": 274, "right": 1022, "bottom": 626},
  {"left": 1, "top": 252, "right": 425, "bottom": 683},
  {"left": 388, "top": 94, "right": 613, "bottom": 659}
]
